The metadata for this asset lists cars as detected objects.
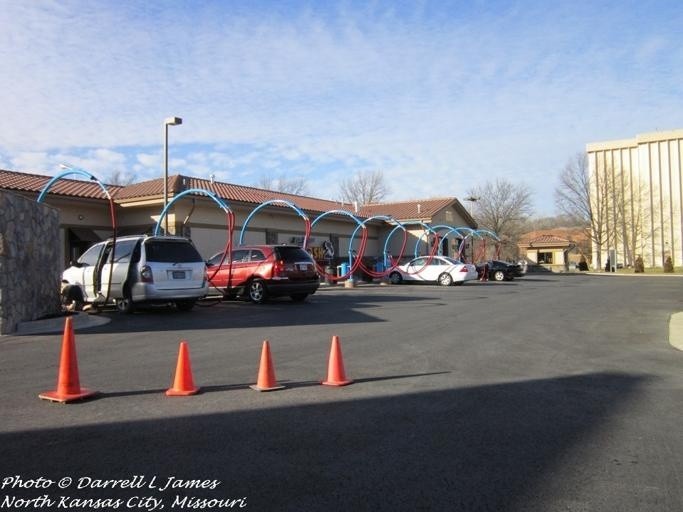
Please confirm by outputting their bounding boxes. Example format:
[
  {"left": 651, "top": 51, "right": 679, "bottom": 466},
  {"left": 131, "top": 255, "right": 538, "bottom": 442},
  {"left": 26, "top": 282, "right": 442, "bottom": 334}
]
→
[
  {"left": 386, "top": 255, "right": 523, "bottom": 286},
  {"left": 60, "top": 234, "right": 320, "bottom": 313}
]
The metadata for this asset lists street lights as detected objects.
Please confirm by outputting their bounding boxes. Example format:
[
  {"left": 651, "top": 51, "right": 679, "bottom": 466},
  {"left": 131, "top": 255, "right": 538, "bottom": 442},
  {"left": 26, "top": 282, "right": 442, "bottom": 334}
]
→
[
  {"left": 463, "top": 196, "right": 480, "bottom": 218},
  {"left": 163, "top": 117, "right": 182, "bottom": 236}
]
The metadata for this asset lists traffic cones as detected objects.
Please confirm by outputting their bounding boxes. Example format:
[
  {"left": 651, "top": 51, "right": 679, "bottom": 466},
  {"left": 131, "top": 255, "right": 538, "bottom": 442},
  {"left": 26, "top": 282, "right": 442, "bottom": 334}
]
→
[
  {"left": 38, "top": 316, "right": 100, "bottom": 405},
  {"left": 249, "top": 339, "right": 287, "bottom": 392},
  {"left": 319, "top": 335, "right": 352, "bottom": 386},
  {"left": 165, "top": 340, "right": 202, "bottom": 397}
]
